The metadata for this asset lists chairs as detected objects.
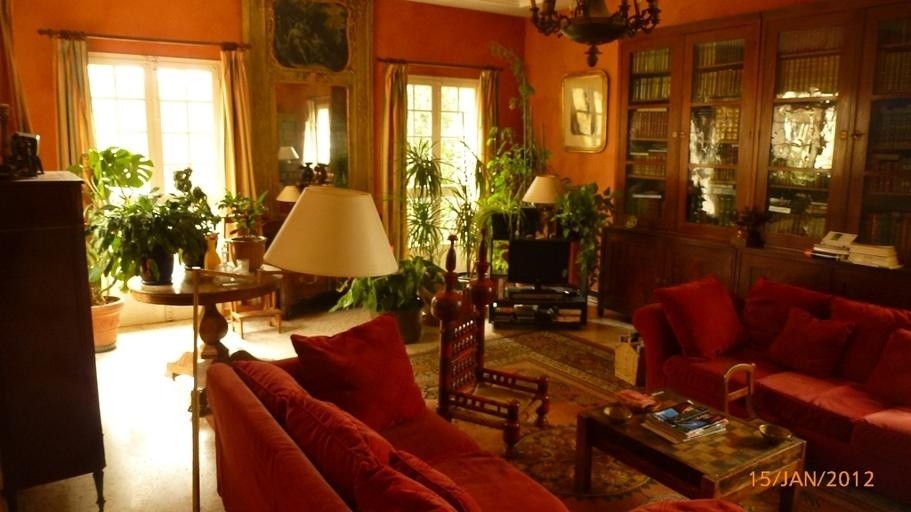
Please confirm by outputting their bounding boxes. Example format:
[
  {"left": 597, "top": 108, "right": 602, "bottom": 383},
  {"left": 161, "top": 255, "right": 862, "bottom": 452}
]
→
[
  {"left": 722, "top": 361, "right": 772, "bottom": 430},
  {"left": 430, "top": 227, "right": 550, "bottom": 462}
]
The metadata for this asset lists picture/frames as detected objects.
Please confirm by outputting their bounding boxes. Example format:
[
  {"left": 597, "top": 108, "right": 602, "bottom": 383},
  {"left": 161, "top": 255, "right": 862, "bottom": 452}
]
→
[{"left": 559, "top": 69, "right": 608, "bottom": 155}]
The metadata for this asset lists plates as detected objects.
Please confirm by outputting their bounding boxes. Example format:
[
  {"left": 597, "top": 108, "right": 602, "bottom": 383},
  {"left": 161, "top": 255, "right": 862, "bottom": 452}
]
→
[{"left": 619, "top": 390, "right": 663, "bottom": 412}]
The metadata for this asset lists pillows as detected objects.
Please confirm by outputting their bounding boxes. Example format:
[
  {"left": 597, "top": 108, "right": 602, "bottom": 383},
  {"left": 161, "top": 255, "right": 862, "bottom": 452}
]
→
[{"left": 648, "top": 273, "right": 911, "bottom": 411}]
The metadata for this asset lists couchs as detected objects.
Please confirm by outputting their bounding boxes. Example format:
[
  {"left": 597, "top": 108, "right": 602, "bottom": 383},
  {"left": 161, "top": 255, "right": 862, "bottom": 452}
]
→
[
  {"left": 203, "top": 312, "right": 570, "bottom": 511},
  {"left": 631, "top": 270, "right": 911, "bottom": 512}
]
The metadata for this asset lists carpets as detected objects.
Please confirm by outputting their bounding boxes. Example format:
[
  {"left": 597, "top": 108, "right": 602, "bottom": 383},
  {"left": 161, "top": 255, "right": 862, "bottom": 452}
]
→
[{"left": 402, "top": 330, "right": 911, "bottom": 512}]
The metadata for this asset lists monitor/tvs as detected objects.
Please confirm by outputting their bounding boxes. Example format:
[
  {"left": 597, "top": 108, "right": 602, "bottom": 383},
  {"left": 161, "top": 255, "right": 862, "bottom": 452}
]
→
[{"left": 507, "top": 239, "right": 570, "bottom": 295}]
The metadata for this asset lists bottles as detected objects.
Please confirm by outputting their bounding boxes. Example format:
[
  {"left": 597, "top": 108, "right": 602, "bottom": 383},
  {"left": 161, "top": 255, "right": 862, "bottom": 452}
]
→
[{"left": 203, "top": 233, "right": 220, "bottom": 277}]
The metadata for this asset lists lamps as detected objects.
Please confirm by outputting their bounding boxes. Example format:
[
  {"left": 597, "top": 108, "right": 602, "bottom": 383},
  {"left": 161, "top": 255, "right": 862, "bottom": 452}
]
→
[
  {"left": 276, "top": 185, "right": 301, "bottom": 211},
  {"left": 530, "top": 0, "right": 661, "bottom": 67},
  {"left": 263, "top": 184, "right": 399, "bottom": 310},
  {"left": 276, "top": 145, "right": 300, "bottom": 184}
]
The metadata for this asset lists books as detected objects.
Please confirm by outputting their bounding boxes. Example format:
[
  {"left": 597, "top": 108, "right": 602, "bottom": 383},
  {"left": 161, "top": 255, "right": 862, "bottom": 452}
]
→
[
  {"left": 686, "top": 38, "right": 745, "bottom": 228},
  {"left": 640, "top": 398, "right": 731, "bottom": 443},
  {"left": 765, "top": 27, "right": 843, "bottom": 237},
  {"left": 629, "top": 47, "right": 670, "bottom": 176},
  {"left": 861, "top": 50, "right": 911, "bottom": 262}
]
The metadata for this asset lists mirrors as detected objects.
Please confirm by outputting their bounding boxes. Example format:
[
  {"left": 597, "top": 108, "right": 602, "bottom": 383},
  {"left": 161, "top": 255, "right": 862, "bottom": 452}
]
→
[{"left": 238, "top": 1, "right": 375, "bottom": 228}]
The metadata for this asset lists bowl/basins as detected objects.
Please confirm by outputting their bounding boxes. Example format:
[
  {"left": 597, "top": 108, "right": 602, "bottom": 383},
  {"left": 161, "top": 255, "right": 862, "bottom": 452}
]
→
[
  {"left": 604, "top": 405, "right": 633, "bottom": 425},
  {"left": 759, "top": 422, "right": 794, "bottom": 446}
]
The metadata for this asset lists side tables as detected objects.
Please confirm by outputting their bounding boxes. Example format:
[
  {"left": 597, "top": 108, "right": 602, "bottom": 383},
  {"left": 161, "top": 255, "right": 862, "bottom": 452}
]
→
[{"left": 127, "top": 263, "right": 285, "bottom": 417}]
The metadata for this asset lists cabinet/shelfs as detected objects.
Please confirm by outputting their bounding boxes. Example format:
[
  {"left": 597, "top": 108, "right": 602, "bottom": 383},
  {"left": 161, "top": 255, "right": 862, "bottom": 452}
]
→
[{"left": 0, "top": 170, "right": 108, "bottom": 512}]
